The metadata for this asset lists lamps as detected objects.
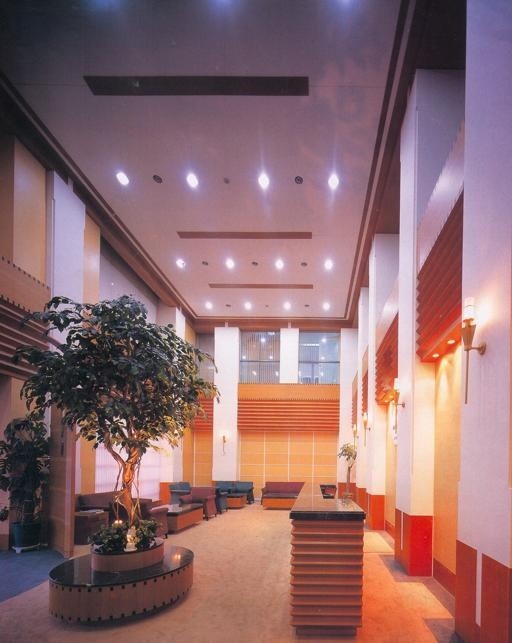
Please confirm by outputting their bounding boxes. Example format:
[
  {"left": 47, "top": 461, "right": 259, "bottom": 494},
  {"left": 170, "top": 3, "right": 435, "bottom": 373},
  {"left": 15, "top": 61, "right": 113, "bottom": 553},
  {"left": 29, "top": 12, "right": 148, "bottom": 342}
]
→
[{"left": 461, "top": 297, "right": 487, "bottom": 404}]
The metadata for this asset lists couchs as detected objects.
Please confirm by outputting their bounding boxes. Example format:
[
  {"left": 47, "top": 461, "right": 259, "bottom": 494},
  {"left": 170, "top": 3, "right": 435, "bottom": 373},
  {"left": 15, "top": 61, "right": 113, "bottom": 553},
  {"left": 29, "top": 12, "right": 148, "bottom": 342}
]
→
[
  {"left": 261, "top": 482, "right": 305, "bottom": 504},
  {"left": 169, "top": 481, "right": 254, "bottom": 514},
  {"left": 79, "top": 490, "right": 169, "bottom": 539}
]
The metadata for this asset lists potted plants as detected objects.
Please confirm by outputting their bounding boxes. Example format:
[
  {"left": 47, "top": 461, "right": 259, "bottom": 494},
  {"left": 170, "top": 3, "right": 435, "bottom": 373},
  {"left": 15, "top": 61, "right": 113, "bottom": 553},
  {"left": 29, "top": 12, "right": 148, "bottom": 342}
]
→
[{"left": 0, "top": 418, "right": 52, "bottom": 548}]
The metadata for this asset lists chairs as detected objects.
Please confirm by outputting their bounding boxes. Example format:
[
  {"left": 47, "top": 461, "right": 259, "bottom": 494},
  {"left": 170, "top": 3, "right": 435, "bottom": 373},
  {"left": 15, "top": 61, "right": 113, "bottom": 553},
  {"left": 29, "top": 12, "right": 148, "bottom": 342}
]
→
[{"left": 179, "top": 487, "right": 217, "bottom": 521}]
